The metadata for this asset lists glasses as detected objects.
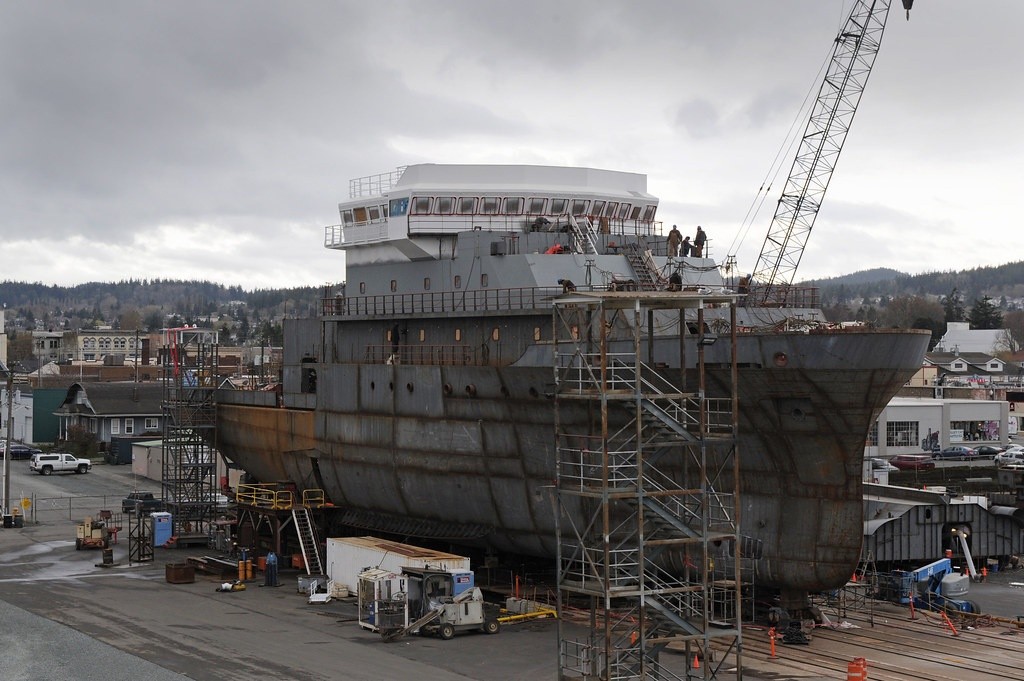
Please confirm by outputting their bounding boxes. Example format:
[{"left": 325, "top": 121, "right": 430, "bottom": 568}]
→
[{"left": 747, "top": 277, "right": 749, "bottom": 279}]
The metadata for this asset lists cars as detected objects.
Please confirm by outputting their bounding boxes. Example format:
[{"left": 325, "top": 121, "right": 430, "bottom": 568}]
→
[{"left": 0, "top": 440, "right": 42, "bottom": 460}]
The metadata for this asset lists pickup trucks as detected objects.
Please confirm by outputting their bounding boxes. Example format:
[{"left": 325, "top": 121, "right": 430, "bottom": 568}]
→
[{"left": 122, "top": 491, "right": 161, "bottom": 514}]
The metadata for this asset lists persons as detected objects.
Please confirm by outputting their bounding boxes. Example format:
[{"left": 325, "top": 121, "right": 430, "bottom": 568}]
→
[
  {"left": 693, "top": 226, "right": 706, "bottom": 257},
  {"left": 973, "top": 428, "right": 994, "bottom": 441},
  {"left": 668, "top": 272, "right": 682, "bottom": 291},
  {"left": 535, "top": 217, "right": 550, "bottom": 232},
  {"left": 558, "top": 279, "right": 577, "bottom": 308},
  {"left": 738, "top": 274, "right": 752, "bottom": 300},
  {"left": 679, "top": 236, "right": 694, "bottom": 257},
  {"left": 667, "top": 225, "right": 682, "bottom": 259}
]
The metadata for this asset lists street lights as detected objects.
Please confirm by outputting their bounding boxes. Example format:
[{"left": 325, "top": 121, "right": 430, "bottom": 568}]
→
[{"left": 0, "top": 361, "right": 30, "bottom": 514}]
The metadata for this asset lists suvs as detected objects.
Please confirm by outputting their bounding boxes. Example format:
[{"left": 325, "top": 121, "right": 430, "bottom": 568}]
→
[{"left": 30, "top": 453, "right": 92, "bottom": 476}]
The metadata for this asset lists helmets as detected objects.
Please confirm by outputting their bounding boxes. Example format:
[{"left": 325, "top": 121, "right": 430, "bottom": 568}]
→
[
  {"left": 747, "top": 274, "right": 751, "bottom": 278},
  {"left": 558, "top": 279, "right": 561, "bottom": 284},
  {"left": 697, "top": 226, "right": 701, "bottom": 230},
  {"left": 673, "top": 225, "right": 677, "bottom": 228}
]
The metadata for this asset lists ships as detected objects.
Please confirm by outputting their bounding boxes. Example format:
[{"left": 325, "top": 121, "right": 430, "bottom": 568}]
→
[{"left": 166, "top": 165, "right": 933, "bottom": 616}]
